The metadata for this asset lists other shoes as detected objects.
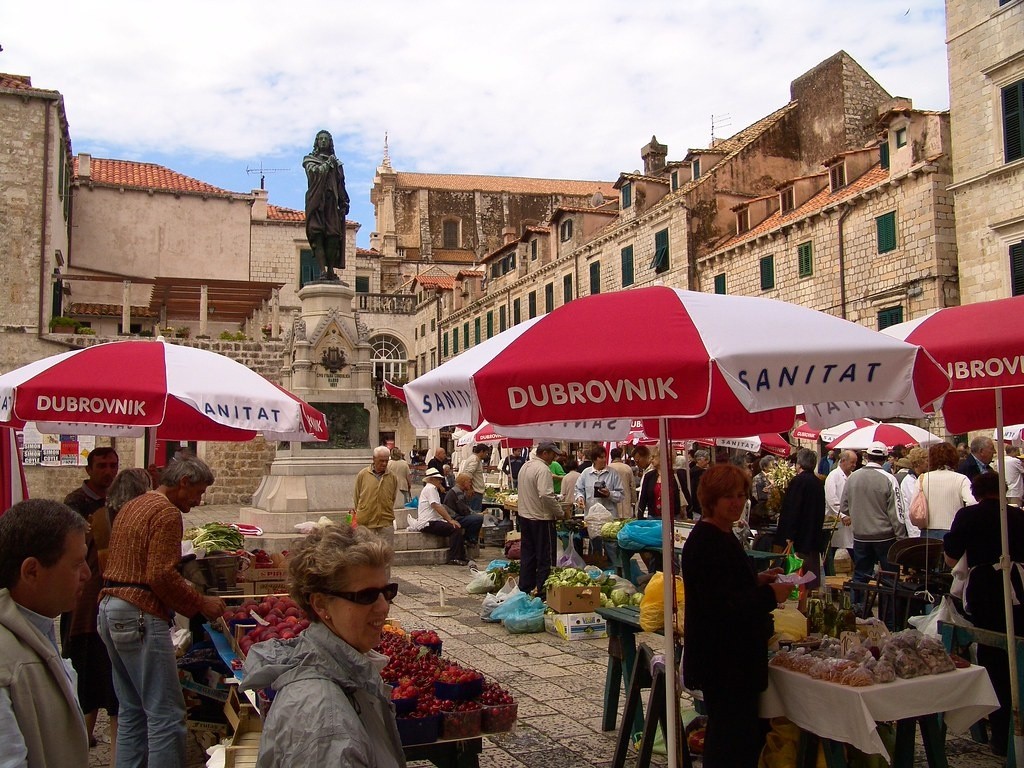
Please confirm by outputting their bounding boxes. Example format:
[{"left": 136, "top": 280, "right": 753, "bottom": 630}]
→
[
  {"left": 463, "top": 540, "right": 485, "bottom": 551},
  {"left": 450, "top": 559, "right": 468, "bottom": 567}
]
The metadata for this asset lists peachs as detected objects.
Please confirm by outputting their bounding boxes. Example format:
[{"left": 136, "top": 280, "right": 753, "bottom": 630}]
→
[{"left": 224, "top": 596, "right": 309, "bottom": 656}]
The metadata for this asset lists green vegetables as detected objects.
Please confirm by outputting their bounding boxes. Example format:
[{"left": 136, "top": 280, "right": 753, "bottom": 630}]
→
[
  {"left": 184, "top": 521, "right": 245, "bottom": 554},
  {"left": 486, "top": 485, "right": 518, "bottom": 497},
  {"left": 488, "top": 559, "right": 616, "bottom": 633}
]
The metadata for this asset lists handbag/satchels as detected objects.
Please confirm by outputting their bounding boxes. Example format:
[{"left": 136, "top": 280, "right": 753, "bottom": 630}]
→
[
  {"left": 909, "top": 473, "right": 929, "bottom": 530},
  {"left": 468, "top": 569, "right": 546, "bottom": 632},
  {"left": 559, "top": 532, "right": 586, "bottom": 569}
]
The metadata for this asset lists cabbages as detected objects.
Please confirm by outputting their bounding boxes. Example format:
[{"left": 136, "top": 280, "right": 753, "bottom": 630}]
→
[
  {"left": 600, "top": 589, "right": 644, "bottom": 608},
  {"left": 601, "top": 521, "right": 622, "bottom": 538}
]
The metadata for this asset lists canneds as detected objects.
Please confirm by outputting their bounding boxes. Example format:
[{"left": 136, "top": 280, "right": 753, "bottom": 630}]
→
[{"left": 778, "top": 640, "right": 795, "bottom": 652}]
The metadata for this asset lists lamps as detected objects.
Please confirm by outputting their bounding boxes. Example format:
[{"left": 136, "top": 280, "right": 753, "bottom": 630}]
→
[{"left": 209, "top": 302, "right": 216, "bottom": 315}]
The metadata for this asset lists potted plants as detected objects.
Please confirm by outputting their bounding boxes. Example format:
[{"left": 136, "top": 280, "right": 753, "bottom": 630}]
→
[
  {"left": 50, "top": 317, "right": 83, "bottom": 334},
  {"left": 178, "top": 325, "right": 191, "bottom": 338}
]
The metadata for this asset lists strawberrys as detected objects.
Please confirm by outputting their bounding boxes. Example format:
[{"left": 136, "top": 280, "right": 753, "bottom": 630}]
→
[{"left": 392, "top": 669, "right": 480, "bottom": 701}]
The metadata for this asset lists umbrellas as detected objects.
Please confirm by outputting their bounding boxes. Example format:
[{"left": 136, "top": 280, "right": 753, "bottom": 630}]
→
[
  {"left": 877, "top": 292, "right": 1024, "bottom": 768},
  {"left": 993, "top": 423, "right": 1024, "bottom": 448},
  {"left": 605, "top": 420, "right": 791, "bottom": 468},
  {"left": 0, "top": 427, "right": 29, "bottom": 518},
  {"left": 0, "top": 334, "right": 329, "bottom": 467},
  {"left": 456, "top": 421, "right": 539, "bottom": 491},
  {"left": 384, "top": 278, "right": 953, "bottom": 768},
  {"left": 789, "top": 416, "right": 878, "bottom": 443},
  {"left": 825, "top": 420, "right": 944, "bottom": 452}
]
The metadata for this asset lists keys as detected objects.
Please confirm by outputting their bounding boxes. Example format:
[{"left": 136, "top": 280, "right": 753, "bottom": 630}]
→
[{"left": 139, "top": 626, "right": 147, "bottom": 645}]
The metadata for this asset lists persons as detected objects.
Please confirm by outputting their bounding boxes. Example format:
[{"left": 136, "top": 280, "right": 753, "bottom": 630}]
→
[
  {"left": 354, "top": 445, "right": 398, "bottom": 583},
  {"left": 302, "top": 128, "right": 350, "bottom": 287},
  {"left": 0, "top": 499, "right": 92, "bottom": 767},
  {"left": 1003, "top": 445, "right": 1024, "bottom": 509},
  {"left": 681, "top": 465, "right": 795, "bottom": 768},
  {"left": 410, "top": 445, "right": 423, "bottom": 465},
  {"left": 236, "top": 523, "right": 410, "bottom": 767},
  {"left": 417, "top": 443, "right": 490, "bottom": 567},
  {"left": 954, "top": 436, "right": 996, "bottom": 484},
  {"left": 733, "top": 440, "right": 978, "bottom": 627},
  {"left": 386, "top": 447, "right": 413, "bottom": 504},
  {"left": 516, "top": 439, "right": 565, "bottom": 604},
  {"left": 547, "top": 442, "right": 713, "bottom": 578},
  {"left": 498, "top": 447, "right": 526, "bottom": 491},
  {"left": 942, "top": 472, "right": 1024, "bottom": 757},
  {"left": 61, "top": 448, "right": 230, "bottom": 768}
]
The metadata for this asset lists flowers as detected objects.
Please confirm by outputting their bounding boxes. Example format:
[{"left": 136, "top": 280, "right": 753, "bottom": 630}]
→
[{"left": 262, "top": 324, "right": 282, "bottom": 335}]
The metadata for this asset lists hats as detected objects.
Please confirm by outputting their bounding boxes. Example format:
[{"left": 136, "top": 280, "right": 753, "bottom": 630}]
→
[
  {"left": 539, "top": 437, "right": 562, "bottom": 455},
  {"left": 422, "top": 467, "right": 444, "bottom": 482},
  {"left": 866, "top": 440, "right": 889, "bottom": 456}
]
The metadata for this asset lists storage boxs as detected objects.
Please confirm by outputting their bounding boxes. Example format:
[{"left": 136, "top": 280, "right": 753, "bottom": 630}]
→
[
  {"left": 175, "top": 553, "right": 518, "bottom": 768},
  {"left": 493, "top": 491, "right": 694, "bottom": 640}
]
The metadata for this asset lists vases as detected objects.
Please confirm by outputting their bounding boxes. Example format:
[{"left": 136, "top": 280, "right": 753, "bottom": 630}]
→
[{"left": 266, "top": 334, "right": 272, "bottom": 338}]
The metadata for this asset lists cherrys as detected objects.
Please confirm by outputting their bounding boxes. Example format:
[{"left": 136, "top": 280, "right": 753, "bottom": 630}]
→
[
  {"left": 405, "top": 677, "right": 517, "bottom": 737},
  {"left": 371, "top": 634, "right": 483, "bottom": 677}
]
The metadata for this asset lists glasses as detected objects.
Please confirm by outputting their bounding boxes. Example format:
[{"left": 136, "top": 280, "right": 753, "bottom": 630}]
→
[
  {"left": 633, "top": 457, "right": 643, "bottom": 464},
  {"left": 318, "top": 582, "right": 399, "bottom": 605}
]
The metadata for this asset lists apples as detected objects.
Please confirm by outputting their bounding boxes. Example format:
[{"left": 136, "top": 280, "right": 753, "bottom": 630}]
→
[{"left": 411, "top": 630, "right": 441, "bottom": 643}]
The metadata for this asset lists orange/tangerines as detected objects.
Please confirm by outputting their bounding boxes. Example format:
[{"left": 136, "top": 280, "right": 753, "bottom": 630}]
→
[{"left": 382, "top": 625, "right": 405, "bottom": 635}]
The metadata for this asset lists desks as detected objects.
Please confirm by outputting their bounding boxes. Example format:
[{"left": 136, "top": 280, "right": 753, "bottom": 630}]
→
[
  {"left": 578, "top": 530, "right": 786, "bottom": 586},
  {"left": 593, "top": 604, "right": 1001, "bottom": 768},
  {"left": 200, "top": 623, "right": 481, "bottom": 768}
]
